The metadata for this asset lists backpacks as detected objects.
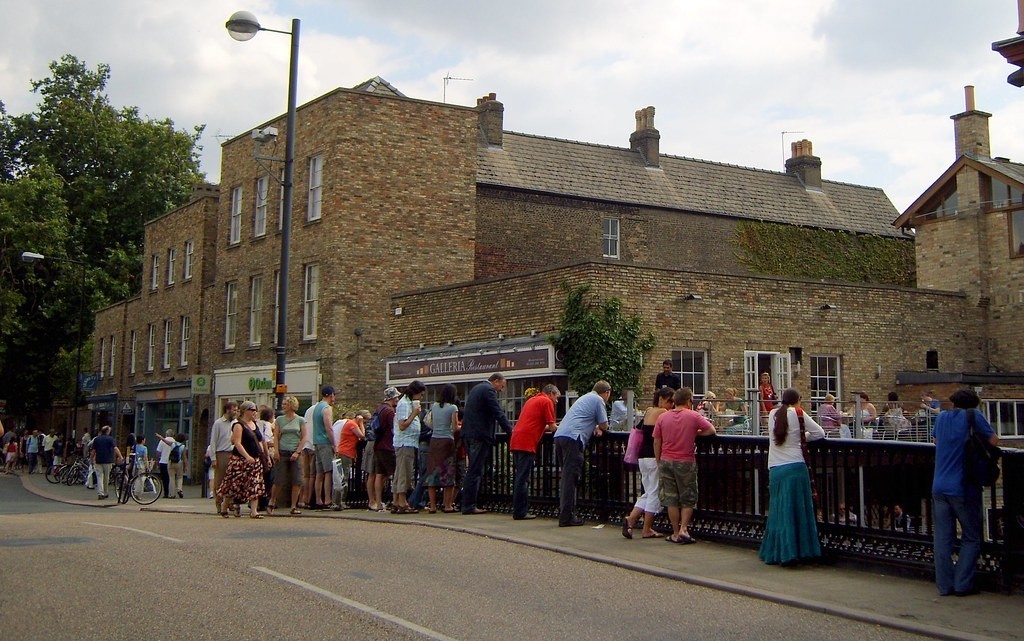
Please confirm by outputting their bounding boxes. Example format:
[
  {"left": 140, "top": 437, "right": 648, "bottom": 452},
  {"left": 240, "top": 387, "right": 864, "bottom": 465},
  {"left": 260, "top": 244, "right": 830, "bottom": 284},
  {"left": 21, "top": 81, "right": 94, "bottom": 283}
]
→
[
  {"left": 169, "top": 442, "right": 183, "bottom": 463},
  {"left": 365, "top": 406, "right": 388, "bottom": 441}
]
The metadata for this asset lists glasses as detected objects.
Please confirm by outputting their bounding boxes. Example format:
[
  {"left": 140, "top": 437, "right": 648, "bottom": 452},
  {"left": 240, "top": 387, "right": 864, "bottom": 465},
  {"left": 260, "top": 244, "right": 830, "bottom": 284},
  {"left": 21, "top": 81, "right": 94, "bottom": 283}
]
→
[{"left": 247, "top": 408, "right": 256, "bottom": 411}]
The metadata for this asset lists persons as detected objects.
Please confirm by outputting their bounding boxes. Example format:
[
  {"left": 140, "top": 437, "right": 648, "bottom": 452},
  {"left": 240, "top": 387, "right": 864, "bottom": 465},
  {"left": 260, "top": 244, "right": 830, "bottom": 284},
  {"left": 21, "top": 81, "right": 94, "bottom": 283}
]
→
[
  {"left": 552, "top": 381, "right": 611, "bottom": 527},
  {"left": 759, "top": 388, "right": 826, "bottom": 567},
  {"left": 653, "top": 386, "right": 716, "bottom": 543},
  {"left": 818, "top": 392, "right": 940, "bottom": 433},
  {"left": 459, "top": 374, "right": 511, "bottom": 515},
  {"left": 623, "top": 387, "right": 675, "bottom": 539},
  {"left": 155, "top": 429, "right": 188, "bottom": 498},
  {"left": 205, "top": 386, "right": 373, "bottom": 518},
  {"left": 133, "top": 435, "right": 150, "bottom": 500},
  {"left": 696, "top": 387, "right": 749, "bottom": 434},
  {"left": 366, "top": 380, "right": 461, "bottom": 513},
  {"left": 932, "top": 389, "right": 998, "bottom": 596},
  {"left": 0, "top": 421, "right": 77, "bottom": 476},
  {"left": 510, "top": 383, "right": 560, "bottom": 520},
  {"left": 759, "top": 371, "right": 779, "bottom": 436},
  {"left": 80, "top": 425, "right": 125, "bottom": 499},
  {"left": 656, "top": 359, "right": 680, "bottom": 391},
  {"left": 816, "top": 503, "right": 911, "bottom": 531},
  {"left": 611, "top": 388, "right": 642, "bottom": 429}
]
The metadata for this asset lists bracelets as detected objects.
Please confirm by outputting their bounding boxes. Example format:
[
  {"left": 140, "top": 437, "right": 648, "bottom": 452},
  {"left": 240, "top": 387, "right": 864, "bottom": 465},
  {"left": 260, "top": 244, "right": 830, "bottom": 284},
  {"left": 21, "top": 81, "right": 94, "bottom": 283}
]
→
[{"left": 298, "top": 452, "right": 300, "bottom": 454}]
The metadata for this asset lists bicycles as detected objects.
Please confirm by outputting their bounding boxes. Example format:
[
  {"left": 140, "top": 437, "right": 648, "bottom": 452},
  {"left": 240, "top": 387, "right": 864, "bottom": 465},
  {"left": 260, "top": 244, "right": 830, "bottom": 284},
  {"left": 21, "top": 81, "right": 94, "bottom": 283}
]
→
[
  {"left": 45, "top": 457, "right": 118, "bottom": 486},
  {"left": 115, "top": 454, "right": 163, "bottom": 505}
]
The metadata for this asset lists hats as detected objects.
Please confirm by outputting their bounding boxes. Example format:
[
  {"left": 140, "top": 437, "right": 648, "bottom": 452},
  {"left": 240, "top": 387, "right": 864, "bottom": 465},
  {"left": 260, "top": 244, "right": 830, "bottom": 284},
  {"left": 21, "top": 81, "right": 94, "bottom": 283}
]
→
[
  {"left": 322, "top": 386, "right": 336, "bottom": 395},
  {"left": 383, "top": 387, "right": 402, "bottom": 402}
]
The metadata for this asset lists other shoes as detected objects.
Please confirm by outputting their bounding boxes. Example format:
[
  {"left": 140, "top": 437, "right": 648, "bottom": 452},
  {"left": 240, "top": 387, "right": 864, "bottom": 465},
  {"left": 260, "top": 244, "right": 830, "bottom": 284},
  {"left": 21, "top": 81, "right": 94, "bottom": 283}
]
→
[
  {"left": 942, "top": 589, "right": 981, "bottom": 596},
  {"left": 98, "top": 494, "right": 108, "bottom": 499},
  {"left": 368, "top": 498, "right": 586, "bottom": 527},
  {"left": 209, "top": 490, "right": 349, "bottom": 518},
  {"left": 134, "top": 493, "right": 141, "bottom": 501},
  {"left": 162, "top": 490, "right": 185, "bottom": 499}
]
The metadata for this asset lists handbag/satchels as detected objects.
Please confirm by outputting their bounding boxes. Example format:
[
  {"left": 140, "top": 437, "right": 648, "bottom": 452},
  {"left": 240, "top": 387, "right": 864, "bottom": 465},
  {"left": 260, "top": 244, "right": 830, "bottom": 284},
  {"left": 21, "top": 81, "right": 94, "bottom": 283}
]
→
[
  {"left": 260, "top": 453, "right": 270, "bottom": 473},
  {"left": 771, "top": 394, "right": 777, "bottom": 405},
  {"left": 144, "top": 476, "right": 156, "bottom": 494},
  {"left": 202, "top": 457, "right": 212, "bottom": 468},
  {"left": 965, "top": 408, "right": 1001, "bottom": 486},
  {"left": 332, "top": 458, "right": 346, "bottom": 489},
  {"left": 423, "top": 405, "right": 434, "bottom": 430},
  {"left": 87, "top": 465, "right": 99, "bottom": 487},
  {"left": 623, "top": 413, "right": 646, "bottom": 466}
]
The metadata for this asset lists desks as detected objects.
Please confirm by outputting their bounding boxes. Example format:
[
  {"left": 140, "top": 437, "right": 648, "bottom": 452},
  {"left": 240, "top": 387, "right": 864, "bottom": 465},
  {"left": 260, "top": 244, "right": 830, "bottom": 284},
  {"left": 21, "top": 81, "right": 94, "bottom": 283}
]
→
[
  {"left": 715, "top": 414, "right": 744, "bottom": 434},
  {"left": 842, "top": 416, "right": 866, "bottom": 434},
  {"left": 906, "top": 416, "right": 934, "bottom": 442}
]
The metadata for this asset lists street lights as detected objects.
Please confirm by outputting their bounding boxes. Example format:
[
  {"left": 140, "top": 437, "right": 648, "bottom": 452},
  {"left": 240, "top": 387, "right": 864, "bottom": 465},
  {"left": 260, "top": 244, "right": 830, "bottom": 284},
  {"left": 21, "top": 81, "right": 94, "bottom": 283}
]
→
[
  {"left": 22, "top": 251, "right": 87, "bottom": 455},
  {"left": 225, "top": 10, "right": 300, "bottom": 418}
]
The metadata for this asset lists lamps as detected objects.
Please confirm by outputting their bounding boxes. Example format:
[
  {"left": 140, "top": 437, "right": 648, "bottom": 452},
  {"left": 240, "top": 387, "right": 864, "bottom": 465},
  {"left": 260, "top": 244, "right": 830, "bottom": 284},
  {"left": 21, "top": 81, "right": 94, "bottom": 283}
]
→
[
  {"left": 820, "top": 304, "right": 837, "bottom": 309},
  {"left": 685, "top": 295, "right": 702, "bottom": 301}
]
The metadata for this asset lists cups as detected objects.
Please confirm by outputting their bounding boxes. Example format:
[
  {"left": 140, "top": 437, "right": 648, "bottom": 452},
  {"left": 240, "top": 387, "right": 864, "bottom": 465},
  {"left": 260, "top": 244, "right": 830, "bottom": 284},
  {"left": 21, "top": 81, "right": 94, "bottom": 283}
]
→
[
  {"left": 919, "top": 410, "right": 925, "bottom": 416},
  {"left": 726, "top": 409, "right": 731, "bottom": 414},
  {"left": 412, "top": 400, "right": 420, "bottom": 408}
]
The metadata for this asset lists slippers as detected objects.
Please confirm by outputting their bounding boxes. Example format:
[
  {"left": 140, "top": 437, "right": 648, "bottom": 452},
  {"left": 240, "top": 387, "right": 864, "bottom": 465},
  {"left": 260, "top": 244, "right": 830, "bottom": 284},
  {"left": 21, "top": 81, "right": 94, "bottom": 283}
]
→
[
  {"left": 679, "top": 534, "right": 696, "bottom": 543},
  {"left": 665, "top": 536, "right": 684, "bottom": 543},
  {"left": 643, "top": 530, "right": 664, "bottom": 538},
  {"left": 622, "top": 517, "right": 632, "bottom": 539}
]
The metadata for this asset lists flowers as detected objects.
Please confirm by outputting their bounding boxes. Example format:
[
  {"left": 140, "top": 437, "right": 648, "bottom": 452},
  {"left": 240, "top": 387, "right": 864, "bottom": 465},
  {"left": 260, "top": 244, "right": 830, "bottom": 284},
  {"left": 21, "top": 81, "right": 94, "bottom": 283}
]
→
[{"left": 524, "top": 387, "right": 540, "bottom": 400}]
